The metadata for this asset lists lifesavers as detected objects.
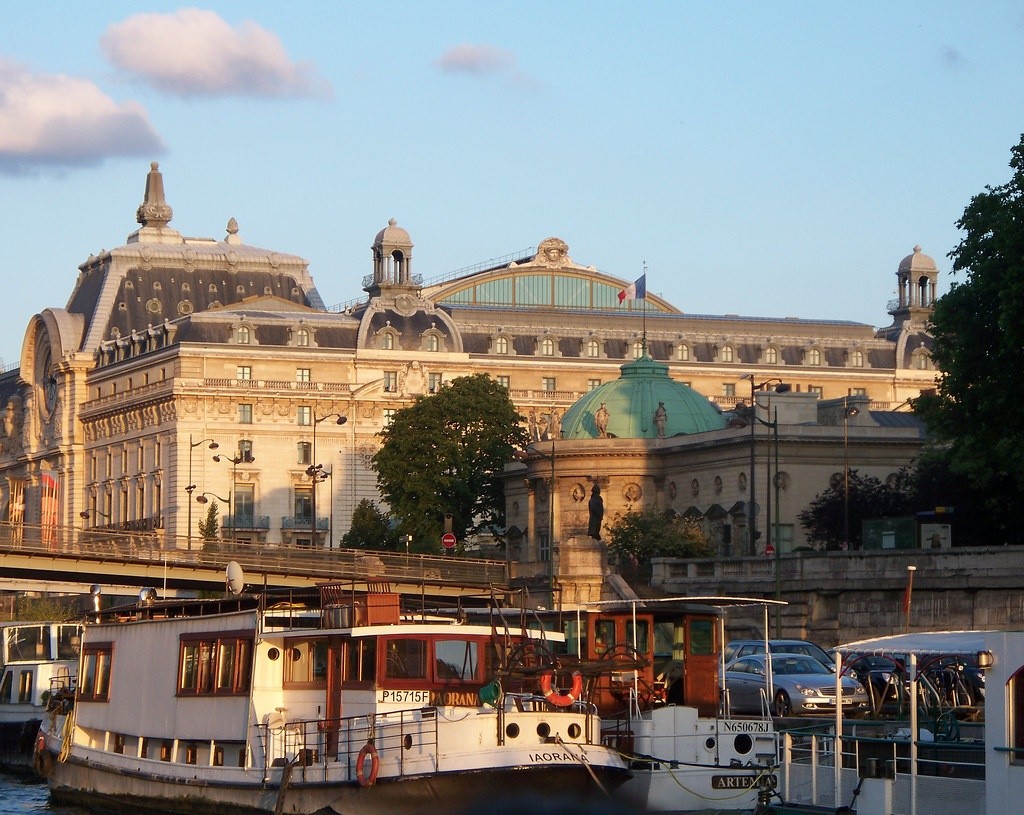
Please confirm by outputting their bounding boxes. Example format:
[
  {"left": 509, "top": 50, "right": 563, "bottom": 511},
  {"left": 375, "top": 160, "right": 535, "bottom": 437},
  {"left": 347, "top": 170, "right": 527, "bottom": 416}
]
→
[
  {"left": 35, "top": 750, "right": 52, "bottom": 778},
  {"left": 356, "top": 744, "right": 379, "bottom": 788},
  {"left": 34, "top": 733, "right": 44, "bottom": 752},
  {"left": 653, "top": 689, "right": 666, "bottom": 703},
  {"left": 541, "top": 670, "right": 583, "bottom": 706}
]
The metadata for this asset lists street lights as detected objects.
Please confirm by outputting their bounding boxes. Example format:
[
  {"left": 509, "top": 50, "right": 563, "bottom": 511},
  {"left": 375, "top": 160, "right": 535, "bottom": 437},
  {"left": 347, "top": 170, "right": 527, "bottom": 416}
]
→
[
  {"left": 741, "top": 373, "right": 791, "bottom": 557},
  {"left": 510, "top": 440, "right": 556, "bottom": 610},
  {"left": 196, "top": 490, "right": 232, "bottom": 557},
  {"left": 320, "top": 464, "right": 333, "bottom": 552},
  {"left": 213, "top": 452, "right": 255, "bottom": 554},
  {"left": 310, "top": 409, "right": 347, "bottom": 577},
  {"left": 80, "top": 508, "right": 112, "bottom": 531},
  {"left": 844, "top": 402, "right": 860, "bottom": 550},
  {"left": 187, "top": 433, "right": 219, "bottom": 551},
  {"left": 735, "top": 398, "right": 782, "bottom": 561}
]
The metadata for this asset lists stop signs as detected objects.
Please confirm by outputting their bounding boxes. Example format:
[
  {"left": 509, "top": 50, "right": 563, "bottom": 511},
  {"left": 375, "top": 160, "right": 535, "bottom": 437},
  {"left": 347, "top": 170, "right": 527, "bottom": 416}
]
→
[
  {"left": 765, "top": 545, "right": 776, "bottom": 558},
  {"left": 441, "top": 533, "right": 456, "bottom": 549}
]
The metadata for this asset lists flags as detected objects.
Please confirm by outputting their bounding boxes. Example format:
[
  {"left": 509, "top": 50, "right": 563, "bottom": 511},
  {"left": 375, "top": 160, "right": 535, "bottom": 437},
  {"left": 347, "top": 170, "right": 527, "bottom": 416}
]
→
[{"left": 617, "top": 273, "right": 646, "bottom": 304}]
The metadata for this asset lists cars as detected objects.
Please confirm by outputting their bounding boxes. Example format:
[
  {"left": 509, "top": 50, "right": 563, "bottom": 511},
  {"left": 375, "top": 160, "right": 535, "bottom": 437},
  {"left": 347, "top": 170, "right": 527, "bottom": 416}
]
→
[
  {"left": 826, "top": 648, "right": 987, "bottom": 703},
  {"left": 718, "top": 652, "right": 869, "bottom": 718}
]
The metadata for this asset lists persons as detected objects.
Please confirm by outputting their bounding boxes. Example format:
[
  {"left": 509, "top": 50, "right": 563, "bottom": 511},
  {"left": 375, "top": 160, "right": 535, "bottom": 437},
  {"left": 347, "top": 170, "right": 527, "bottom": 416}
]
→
[
  {"left": 654, "top": 401, "right": 667, "bottom": 437},
  {"left": 588, "top": 484, "right": 604, "bottom": 540},
  {"left": 594, "top": 401, "right": 610, "bottom": 439},
  {"left": 538, "top": 238, "right": 569, "bottom": 261},
  {"left": 527, "top": 410, "right": 562, "bottom": 442}
]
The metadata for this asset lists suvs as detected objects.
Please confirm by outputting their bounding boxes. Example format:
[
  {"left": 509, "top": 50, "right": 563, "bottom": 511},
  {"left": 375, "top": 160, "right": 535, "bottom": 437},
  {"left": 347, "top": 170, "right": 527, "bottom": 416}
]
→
[{"left": 716, "top": 639, "right": 858, "bottom": 683}]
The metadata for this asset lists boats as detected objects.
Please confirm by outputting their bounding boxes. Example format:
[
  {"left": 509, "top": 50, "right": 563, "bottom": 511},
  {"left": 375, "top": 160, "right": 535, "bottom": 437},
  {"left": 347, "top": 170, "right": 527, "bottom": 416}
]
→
[{"left": 1, "top": 575, "right": 783, "bottom": 815}]
